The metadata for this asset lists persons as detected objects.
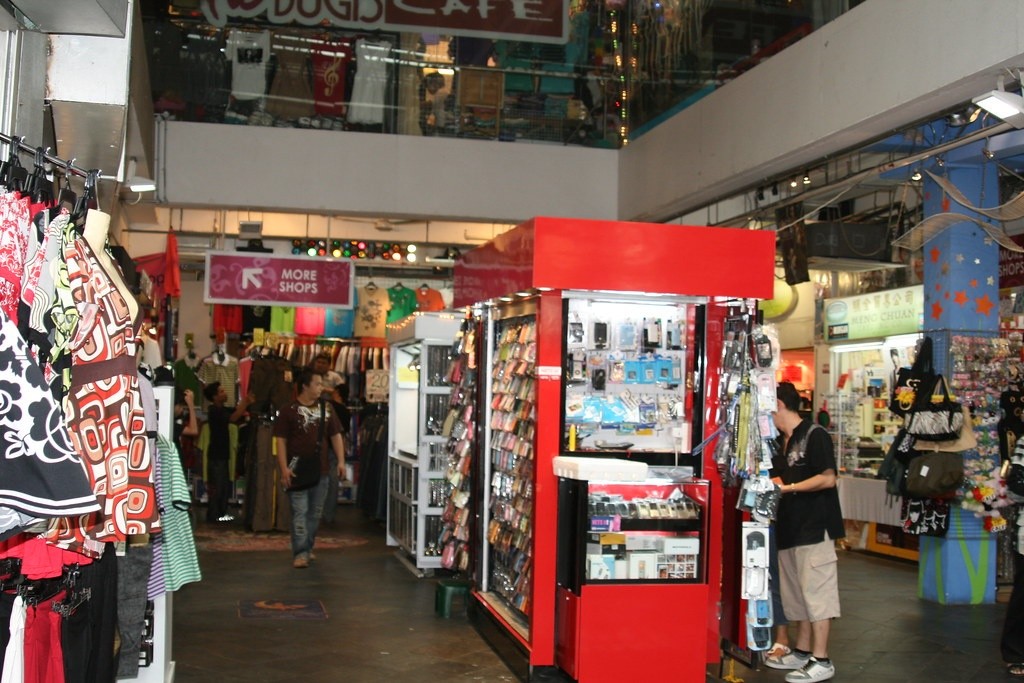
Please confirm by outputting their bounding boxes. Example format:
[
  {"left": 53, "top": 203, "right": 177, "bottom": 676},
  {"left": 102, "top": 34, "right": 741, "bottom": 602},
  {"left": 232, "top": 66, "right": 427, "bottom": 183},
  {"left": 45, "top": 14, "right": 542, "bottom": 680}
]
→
[
  {"left": 1000, "top": 433, "right": 1024, "bottom": 676},
  {"left": 274, "top": 351, "right": 352, "bottom": 568},
  {"left": 890, "top": 349, "right": 905, "bottom": 384},
  {"left": 64, "top": 209, "right": 138, "bottom": 447},
  {"left": 416, "top": 67, "right": 457, "bottom": 129},
  {"left": 203, "top": 381, "right": 256, "bottom": 524},
  {"left": 761, "top": 381, "right": 845, "bottom": 683},
  {"left": 172, "top": 388, "right": 198, "bottom": 452}
]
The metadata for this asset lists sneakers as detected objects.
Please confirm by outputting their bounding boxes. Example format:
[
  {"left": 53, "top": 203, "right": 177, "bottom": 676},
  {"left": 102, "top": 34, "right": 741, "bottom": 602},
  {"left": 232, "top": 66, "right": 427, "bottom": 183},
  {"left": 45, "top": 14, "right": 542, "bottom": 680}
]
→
[
  {"left": 766, "top": 650, "right": 810, "bottom": 669},
  {"left": 785, "top": 657, "right": 835, "bottom": 683}
]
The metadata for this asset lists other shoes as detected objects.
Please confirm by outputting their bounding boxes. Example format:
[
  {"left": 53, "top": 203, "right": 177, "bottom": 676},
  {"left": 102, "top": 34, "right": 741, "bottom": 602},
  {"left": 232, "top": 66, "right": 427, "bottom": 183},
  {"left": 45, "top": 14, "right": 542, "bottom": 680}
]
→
[
  {"left": 309, "top": 553, "right": 317, "bottom": 560},
  {"left": 293, "top": 558, "right": 309, "bottom": 568}
]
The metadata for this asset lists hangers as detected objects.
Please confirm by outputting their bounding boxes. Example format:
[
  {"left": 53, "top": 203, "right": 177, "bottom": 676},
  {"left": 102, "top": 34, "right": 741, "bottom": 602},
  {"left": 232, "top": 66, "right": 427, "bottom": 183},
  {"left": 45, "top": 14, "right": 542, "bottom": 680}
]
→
[
  {"left": 181, "top": 19, "right": 388, "bottom": 45},
  {"left": 243, "top": 334, "right": 388, "bottom": 351},
  {"left": 186, "top": 334, "right": 227, "bottom": 362},
  {"left": 0, "top": 135, "right": 99, "bottom": 218},
  {"left": 344, "top": 405, "right": 387, "bottom": 422},
  {"left": 360, "top": 277, "right": 456, "bottom": 291}
]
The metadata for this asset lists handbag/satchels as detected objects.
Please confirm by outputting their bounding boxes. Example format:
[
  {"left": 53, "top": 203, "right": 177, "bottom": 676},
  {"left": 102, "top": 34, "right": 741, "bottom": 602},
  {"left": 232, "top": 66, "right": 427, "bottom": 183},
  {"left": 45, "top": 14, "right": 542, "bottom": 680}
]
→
[
  {"left": 878, "top": 336, "right": 977, "bottom": 537},
  {"left": 281, "top": 453, "right": 322, "bottom": 491}
]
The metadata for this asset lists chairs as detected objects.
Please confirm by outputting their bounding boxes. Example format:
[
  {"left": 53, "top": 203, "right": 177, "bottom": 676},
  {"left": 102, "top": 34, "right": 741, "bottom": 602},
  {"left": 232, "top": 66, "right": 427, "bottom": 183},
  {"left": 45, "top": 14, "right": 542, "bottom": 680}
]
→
[{"left": 456, "top": 68, "right": 505, "bottom": 139}]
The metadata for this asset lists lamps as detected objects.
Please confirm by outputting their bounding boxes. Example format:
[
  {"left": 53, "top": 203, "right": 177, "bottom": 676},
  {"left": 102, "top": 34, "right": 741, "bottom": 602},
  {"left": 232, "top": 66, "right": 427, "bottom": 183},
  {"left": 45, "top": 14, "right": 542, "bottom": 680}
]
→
[
  {"left": 791, "top": 176, "right": 797, "bottom": 187},
  {"left": 756, "top": 185, "right": 765, "bottom": 200},
  {"left": 948, "top": 112, "right": 966, "bottom": 128},
  {"left": 912, "top": 158, "right": 927, "bottom": 181},
  {"left": 122, "top": 156, "right": 156, "bottom": 193},
  {"left": 972, "top": 89, "right": 1024, "bottom": 130},
  {"left": 935, "top": 156, "right": 944, "bottom": 168},
  {"left": 963, "top": 106, "right": 982, "bottom": 124},
  {"left": 237, "top": 221, "right": 264, "bottom": 240},
  {"left": 772, "top": 183, "right": 779, "bottom": 195},
  {"left": 980, "top": 149, "right": 995, "bottom": 158},
  {"left": 803, "top": 169, "right": 812, "bottom": 184}
]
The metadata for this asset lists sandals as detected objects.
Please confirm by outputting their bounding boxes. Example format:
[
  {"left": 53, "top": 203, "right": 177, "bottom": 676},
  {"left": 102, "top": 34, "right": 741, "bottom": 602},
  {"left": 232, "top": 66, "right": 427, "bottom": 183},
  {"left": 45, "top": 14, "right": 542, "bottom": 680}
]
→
[
  {"left": 1006, "top": 662, "right": 1024, "bottom": 677},
  {"left": 765, "top": 642, "right": 792, "bottom": 658}
]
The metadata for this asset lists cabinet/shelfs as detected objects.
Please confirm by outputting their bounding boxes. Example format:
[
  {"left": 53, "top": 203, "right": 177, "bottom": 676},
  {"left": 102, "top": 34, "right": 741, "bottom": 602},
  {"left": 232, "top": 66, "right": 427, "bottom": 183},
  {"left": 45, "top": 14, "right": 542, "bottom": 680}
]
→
[
  {"left": 872, "top": 395, "right": 906, "bottom": 439},
  {"left": 385, "top": 337, "right": 455, "bottom": 569}
]
